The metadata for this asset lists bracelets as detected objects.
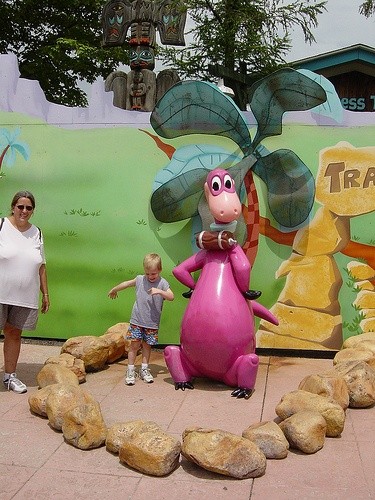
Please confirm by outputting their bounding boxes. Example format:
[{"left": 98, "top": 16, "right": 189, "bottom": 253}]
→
[{"left": 43, "top": 293, "right": 47, "bottom": 296}]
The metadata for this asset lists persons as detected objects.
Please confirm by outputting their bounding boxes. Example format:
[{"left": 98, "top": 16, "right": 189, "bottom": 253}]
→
[
  {"left": 107, "top": 253, "right": 174, "bottom": 386},
  {"left": 0, "top": 191, "right": 51, "bottom": 395}
]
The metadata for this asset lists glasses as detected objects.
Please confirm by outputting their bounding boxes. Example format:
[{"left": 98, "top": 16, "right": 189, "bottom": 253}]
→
[{"left": 15, "top": 204, "right": 34, "bottom": 211}]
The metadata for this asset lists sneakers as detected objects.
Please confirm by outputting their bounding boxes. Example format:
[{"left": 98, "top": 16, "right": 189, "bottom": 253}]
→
[
  {"left": 138, "top": 368, "right": 154, "bottom": 383},
  {"left": 2, "top": 373, "right": 27, "bottom": 394},
  {"left": 124, "top": 366, "right": 137, "bottom": 386}
]
